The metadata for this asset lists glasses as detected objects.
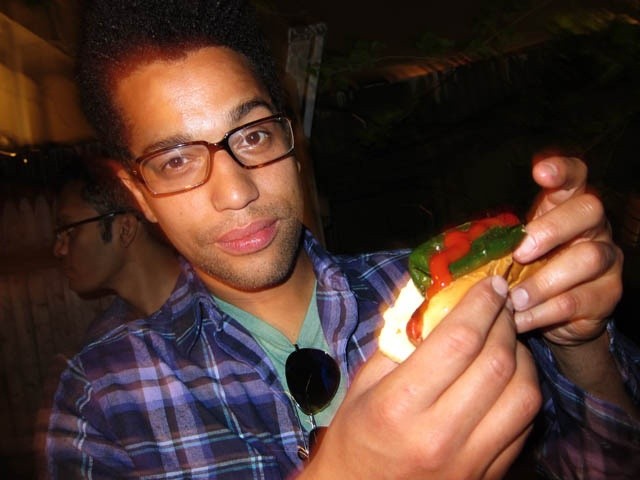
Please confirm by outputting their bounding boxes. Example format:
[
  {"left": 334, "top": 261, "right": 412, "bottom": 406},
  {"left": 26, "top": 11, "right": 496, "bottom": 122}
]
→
[
  {"left": 54, "top": 208, "right": 132, "bottom": 237},
  {"left": 132, "top": 112, "right": 298, "bottom": 199},
  {"left": 285, "top": 344, "right": 341, "bottom": 463}
]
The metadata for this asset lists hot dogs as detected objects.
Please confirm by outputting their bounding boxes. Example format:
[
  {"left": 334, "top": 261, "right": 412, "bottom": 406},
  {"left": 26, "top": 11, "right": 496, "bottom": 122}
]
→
[{"left": 377, "top": 212, "right": 547, "bottom": 365}]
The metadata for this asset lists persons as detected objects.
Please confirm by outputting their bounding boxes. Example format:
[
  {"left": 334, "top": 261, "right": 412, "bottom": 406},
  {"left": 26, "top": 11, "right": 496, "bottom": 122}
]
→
[
  {"left": 43, "top": 5, "right": 640, "bottom": 479},
  {"left": 49, "top": 159, "right": 182, "bottom": 316}
]
